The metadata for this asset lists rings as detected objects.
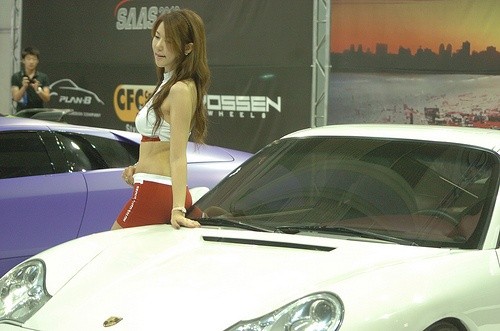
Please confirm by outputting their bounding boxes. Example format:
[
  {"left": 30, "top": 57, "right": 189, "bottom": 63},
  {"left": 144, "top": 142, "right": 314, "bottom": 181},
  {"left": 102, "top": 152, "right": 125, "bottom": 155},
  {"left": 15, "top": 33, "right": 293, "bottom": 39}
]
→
[{"left": 125, "top": 176, "right": 128, "bottom": 178}]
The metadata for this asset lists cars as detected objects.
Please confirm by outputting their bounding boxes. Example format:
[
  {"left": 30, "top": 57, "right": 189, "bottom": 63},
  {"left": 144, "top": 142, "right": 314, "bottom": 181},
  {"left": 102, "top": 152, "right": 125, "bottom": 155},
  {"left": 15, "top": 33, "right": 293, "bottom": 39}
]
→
[
  {"left": 0, "top": 116, "right": 255, "bottom": 278},
  {"left": 0, "top": 124, "right": 500, "bottom": 331}
]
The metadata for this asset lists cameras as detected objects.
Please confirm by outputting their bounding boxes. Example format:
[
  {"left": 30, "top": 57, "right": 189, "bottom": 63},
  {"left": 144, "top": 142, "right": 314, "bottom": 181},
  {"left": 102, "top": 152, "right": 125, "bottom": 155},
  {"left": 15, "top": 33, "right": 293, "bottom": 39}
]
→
[{"left": 29, "top": 75, "right": 38, "bottom": 83}]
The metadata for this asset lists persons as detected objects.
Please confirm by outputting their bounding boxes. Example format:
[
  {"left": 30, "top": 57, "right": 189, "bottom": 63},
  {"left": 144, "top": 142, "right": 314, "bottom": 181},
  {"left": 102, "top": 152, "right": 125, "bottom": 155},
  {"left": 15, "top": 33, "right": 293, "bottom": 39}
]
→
[
  {"left": 10, "top": 47, "right": 51, "bottom": 119},
  {"left": 110, "top": 9, "right": 207, "bottom": 230}
]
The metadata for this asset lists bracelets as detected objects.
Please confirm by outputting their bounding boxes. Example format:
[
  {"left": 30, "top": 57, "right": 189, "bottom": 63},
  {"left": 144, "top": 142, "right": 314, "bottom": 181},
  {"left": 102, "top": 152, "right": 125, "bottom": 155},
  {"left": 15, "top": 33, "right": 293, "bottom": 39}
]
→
[{"left": 36, "top": 87, "right": 42, "bottom": 94}]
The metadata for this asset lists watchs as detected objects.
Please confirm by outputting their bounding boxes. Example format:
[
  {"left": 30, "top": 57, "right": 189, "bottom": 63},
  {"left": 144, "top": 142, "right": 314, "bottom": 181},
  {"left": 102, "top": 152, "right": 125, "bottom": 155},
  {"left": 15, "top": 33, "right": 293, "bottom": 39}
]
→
[{"left": 171, "top": 206, "right": 188, "bottom": 214}]
